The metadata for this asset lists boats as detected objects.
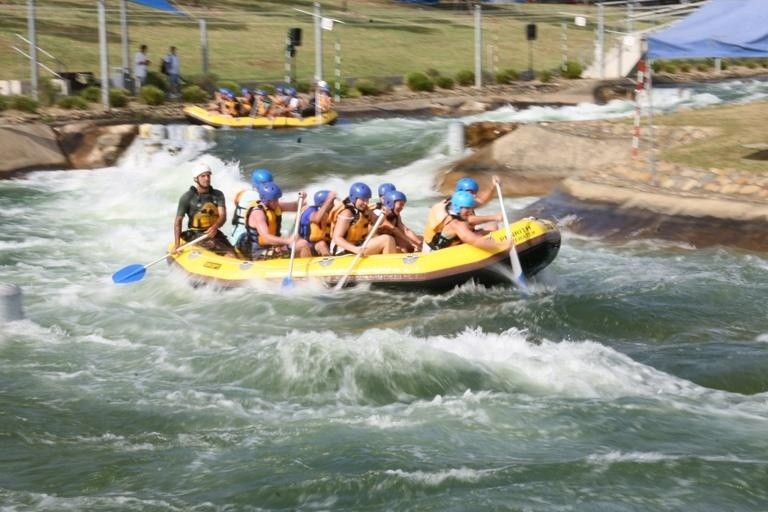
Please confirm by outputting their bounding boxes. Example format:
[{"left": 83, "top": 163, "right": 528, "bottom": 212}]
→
[
  {"left": 167, "top": 216, "right": 562, "bottom": 291},
  {"left": 181, "top": 105, "right": 340, "bottom": 131}
]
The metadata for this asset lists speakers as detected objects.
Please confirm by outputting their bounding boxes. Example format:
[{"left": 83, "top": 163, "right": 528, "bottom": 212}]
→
[
  {"left": 290, "top": 28, "right": 301, "bottom": 45},
  {"left": 527, "top": 24, "right": 534, "bottom": 40}
]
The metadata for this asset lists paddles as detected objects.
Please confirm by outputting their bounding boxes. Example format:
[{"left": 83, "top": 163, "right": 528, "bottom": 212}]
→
[
  {"left": 111, "top": 232, "right": 209, "bottom": 283},
  {"left": 494, "top": 183, "right": 526, "bottom": 290},
  {"left": 279, "top": 197, "right": 303, "bottom": 286}
]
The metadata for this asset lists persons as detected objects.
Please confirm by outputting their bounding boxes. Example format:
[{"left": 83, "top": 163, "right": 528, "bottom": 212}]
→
[
  {"left": 366, "top": 182, "right": 396, "bottom": 217},
  {"left": 232, "top": 166, "right": 274, "bottom": 246},
  {"left": 134, "top": 45, "right": 152, "bottom": 96},
  {"left": 204, "top": 81, "right": 335, "bottom": 119},
  {"left": 169, "top": 160, "right": 238, "bottom": 260},
  {"left": 422, "top": 191, "right": 513, "bottom": 254},
  {"left": 159, "top": 44, "right": 183, "bottom": 99},
  {"left": 425, "top": 174, "right": 503, "bottom": 236},
  {"left": 298, "top": 189, "right": 340, "bottom": 256},
  {"left": 327, "top": 181, "right": 397, "bottom": 257},
  {"left": 366, "top": 190, "right": 424, "bottom": 253},
  {"left": 244, "top": 181, "right": 311, "bottom": 261}
]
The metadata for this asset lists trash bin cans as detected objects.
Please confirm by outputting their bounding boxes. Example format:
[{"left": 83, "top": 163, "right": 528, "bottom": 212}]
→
[{"left": 55, "top": 71, "right": 94, "bottom": 96}]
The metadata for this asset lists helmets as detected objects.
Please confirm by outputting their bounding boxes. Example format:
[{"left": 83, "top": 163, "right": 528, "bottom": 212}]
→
[
  {"left": 450, "top": 192, "right": 474, "bottom": 215},
  {"left": 251, "top": 168, "right": 275, "bottom": 191},
  {"left": 258, "top": 182, "right": 282, "bottom": 205},
  {"left": 317, "top": 79, "right": 330, "bottom": 93},
  {"left": 217, "top": 84, "right": 296, "bottom": 100},
  {"left": 455, "top": 177, "right": 480, "bottom": 193},
  {"left": 314, "top": 182, "right": 408, "bottom": 213},
  {"left": 191, "top": 163, "right": 213, "bottom": 182}
]
